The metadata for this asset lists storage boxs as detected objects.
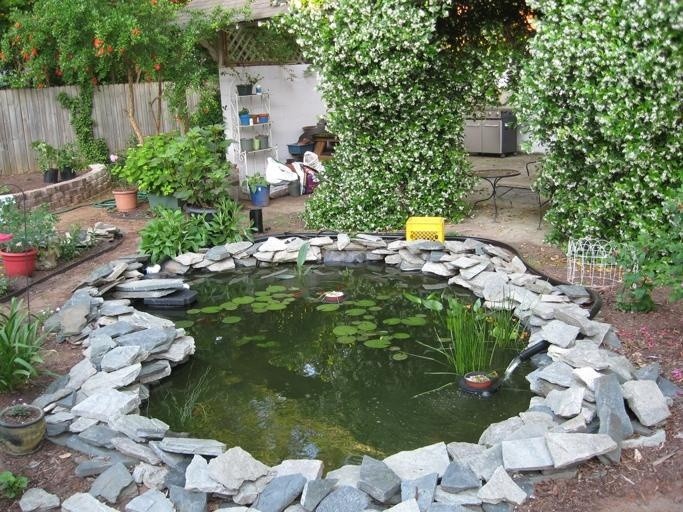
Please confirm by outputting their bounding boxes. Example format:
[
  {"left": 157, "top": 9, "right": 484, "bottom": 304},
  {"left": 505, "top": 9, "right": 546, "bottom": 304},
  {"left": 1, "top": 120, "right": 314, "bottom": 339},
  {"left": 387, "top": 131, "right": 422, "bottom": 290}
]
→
[{"left": 287, "top": 142, "right": 314, "bottom": 155}]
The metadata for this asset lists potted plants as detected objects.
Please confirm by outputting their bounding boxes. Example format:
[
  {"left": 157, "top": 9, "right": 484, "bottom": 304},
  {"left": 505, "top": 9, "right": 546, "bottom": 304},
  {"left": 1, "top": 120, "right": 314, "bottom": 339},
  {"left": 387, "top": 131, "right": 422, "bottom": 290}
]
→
[
  {"left": 127, "top": 133, "right": 182, "bottom": 211},
  {"left": 53, "top": 143, "right": 77, "bottom": 182},
  {"left": 239, "top": 107, "right": 249, "bottom": 125},
  {"left": 150, "top": 122, "right": 235, "bottom": 218},
  {"left": 221, "top": 65, "right": 263, "bottom": 94},
  {"left": 244, "top": 173, "right": 271, "bottom": 207},
  {"left": 30, "top": 140, "right": 59, "bottom": 183}
]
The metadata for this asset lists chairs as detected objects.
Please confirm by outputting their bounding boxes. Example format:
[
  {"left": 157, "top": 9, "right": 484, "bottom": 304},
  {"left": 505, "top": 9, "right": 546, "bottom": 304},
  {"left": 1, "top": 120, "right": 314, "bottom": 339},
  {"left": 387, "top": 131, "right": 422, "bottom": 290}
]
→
[{"left": 492, "top": 159, "right": 551, "bottom": 228}]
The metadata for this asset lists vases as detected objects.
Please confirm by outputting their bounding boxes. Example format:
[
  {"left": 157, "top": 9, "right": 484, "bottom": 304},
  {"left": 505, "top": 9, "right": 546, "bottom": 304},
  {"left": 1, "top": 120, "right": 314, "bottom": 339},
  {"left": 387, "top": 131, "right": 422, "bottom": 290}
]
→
[
  {"left": 258, "top": 113, "right": 269, "bottom": 123},
  {"left": 464, "top": 371, "right": 493, "bottom": 390},
  {"left": 108, "top": 187, "right": 138, "bottom": 211},
  {"left": 0, "top": 247, "right": 37, "bottom": 278},
  {"left": 249, "top": 114, "right": 258, "bottom": 124},
  {"left": 0, "top": 404, "right": 45, "bottom": 457}
]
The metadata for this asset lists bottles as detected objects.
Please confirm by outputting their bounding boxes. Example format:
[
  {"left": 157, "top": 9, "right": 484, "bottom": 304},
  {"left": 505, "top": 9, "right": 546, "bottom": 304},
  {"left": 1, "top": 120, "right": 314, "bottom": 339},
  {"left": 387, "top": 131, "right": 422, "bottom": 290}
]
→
[{"left": 249, "top": 119, "right": 253, "bottom": 125}]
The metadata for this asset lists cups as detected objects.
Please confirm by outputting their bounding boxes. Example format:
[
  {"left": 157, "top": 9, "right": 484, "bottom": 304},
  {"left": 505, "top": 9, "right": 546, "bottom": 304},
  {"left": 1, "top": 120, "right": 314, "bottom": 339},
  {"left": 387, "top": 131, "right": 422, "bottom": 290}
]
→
[
  {"left": 252, "top": 140, "right": 259, "bottom": 150},
  {"left": 256, "top": 85, "right": 261, "bottom": 94}
]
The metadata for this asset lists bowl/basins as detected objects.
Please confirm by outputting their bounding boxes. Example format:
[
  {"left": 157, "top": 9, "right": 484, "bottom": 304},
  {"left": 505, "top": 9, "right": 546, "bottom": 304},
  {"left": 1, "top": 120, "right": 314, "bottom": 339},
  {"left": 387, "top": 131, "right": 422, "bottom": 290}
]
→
[
  {"left": 259, "top": 117, "right": 267, "bottom": 123},
  {"left": 463, "top": 371, "right": 492, "bottom": 388}
]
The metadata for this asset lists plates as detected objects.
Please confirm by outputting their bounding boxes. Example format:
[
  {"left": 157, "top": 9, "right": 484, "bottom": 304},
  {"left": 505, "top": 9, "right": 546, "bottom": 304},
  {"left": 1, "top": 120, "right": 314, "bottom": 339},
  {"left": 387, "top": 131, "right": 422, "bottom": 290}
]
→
[{"left": 257, "top": 114, "right": 268, "bottom": 117}]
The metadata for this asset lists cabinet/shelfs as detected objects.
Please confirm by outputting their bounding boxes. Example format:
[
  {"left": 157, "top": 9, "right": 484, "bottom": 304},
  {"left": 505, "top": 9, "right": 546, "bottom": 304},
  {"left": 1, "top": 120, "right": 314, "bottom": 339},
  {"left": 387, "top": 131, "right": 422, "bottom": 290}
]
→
[
  {"left": 231, "top": 86, "right": 279, "bottom": 175},
  {"left": 459, "top": 107, "right": 516, "bottom": 157}
]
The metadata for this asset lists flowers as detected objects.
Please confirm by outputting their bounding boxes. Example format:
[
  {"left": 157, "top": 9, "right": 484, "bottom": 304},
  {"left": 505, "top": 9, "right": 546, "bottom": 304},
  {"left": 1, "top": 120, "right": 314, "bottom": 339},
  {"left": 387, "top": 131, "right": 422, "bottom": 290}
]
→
[
  {"left": 8, "top": 398, "right": 29, "bottom": 417},
  {"left": 0, "top": 185, "right": 61, "bottom": 253},
  {"left": 107, "top": 151, "right": 133, "bottom": 191}
]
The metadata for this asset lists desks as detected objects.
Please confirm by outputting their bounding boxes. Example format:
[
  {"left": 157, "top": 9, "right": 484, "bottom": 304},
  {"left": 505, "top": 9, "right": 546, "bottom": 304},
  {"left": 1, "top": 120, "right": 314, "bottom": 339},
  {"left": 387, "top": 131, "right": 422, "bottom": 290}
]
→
[
  {"left": 311, "top": 138, "right": 342, "bottom": 158},
  {"left": 469, "top": 168, "right": 520, "bottom": 215}
]
